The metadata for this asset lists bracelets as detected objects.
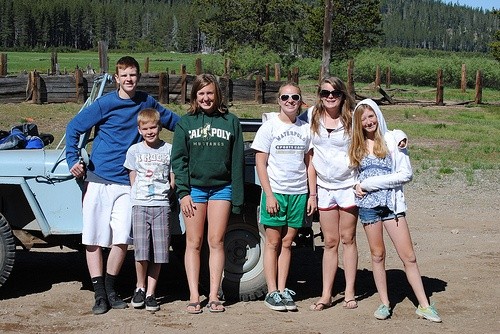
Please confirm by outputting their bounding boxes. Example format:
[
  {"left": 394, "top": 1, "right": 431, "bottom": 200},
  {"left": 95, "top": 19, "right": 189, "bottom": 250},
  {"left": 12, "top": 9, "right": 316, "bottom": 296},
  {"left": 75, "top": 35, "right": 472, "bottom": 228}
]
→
[{"left": 311, "top": 193, "right": 317, "bottom": 196}]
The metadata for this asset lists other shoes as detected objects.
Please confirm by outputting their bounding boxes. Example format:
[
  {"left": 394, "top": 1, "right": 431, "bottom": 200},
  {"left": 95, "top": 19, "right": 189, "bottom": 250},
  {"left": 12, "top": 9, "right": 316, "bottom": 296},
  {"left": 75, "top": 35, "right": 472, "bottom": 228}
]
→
[
  {"left": 92, "top": 296, "right": 109, "bottom": 314},
  {"left": 108, "top": 292, "right": 128, "bottom": 309},
  {"left": 132, "top": 288, "right": 146, "bottom": 308},
  {"left": 145, "top": 295, "right": 161, "bottom": 310}
]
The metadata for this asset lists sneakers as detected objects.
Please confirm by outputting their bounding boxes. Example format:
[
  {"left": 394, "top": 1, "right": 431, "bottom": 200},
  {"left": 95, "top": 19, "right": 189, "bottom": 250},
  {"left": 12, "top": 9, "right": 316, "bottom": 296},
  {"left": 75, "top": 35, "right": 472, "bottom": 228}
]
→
[
  {"left": 281, "top": 288, "right": 297, "bottom": 311},
  {"left": 415, "top": 301, "right": 441, "bottom": 323},
  {"left": 374, "top": 304, "right": 391, "bottom": 320},
  {"left": 264, "top": 290, "right": 286, "bottom": 311}
]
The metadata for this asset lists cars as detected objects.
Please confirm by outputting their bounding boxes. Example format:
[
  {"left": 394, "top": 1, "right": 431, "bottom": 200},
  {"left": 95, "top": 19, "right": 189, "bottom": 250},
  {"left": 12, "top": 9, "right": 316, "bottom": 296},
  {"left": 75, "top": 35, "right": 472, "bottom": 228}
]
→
[{"left": 0, "top": 73, "right": 316, "bottom": 303}]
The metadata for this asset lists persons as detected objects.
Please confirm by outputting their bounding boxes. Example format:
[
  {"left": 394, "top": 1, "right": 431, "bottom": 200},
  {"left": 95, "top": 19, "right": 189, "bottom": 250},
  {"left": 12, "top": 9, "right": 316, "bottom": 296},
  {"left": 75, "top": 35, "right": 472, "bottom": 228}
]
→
[
  {"left": 171, "top": 74, "right": 244, "bottom": 312},
  {"left": 350, "top": 98, "right": 442, "bottom": 322},
  {"left": 298, "top": 76, "right": 408, "bottom": 311},
  {"left": 65, "top": 56, "right": 181, "bottom": 312},
  {"left": 123, "top": 108, "right": 175, "bottom": 310},
  {"left": 250, "top": 82, "right": 318, "bottom": 310}
]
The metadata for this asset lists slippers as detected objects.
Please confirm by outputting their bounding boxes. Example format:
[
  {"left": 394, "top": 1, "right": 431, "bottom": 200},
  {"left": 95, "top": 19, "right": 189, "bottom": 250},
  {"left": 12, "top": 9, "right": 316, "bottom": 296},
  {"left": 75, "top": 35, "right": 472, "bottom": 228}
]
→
[
  {"left": 311, "top": 302, "right": 331, "bottom": 311},
  {"left": 187, "top": 303, "right": 202, "bottom": 313},
  {"left": 344, "top": 300, "right": 356, "bottom": 309},
  {"left": 207, "top": 301, "right": 225, "bottom": 312}
]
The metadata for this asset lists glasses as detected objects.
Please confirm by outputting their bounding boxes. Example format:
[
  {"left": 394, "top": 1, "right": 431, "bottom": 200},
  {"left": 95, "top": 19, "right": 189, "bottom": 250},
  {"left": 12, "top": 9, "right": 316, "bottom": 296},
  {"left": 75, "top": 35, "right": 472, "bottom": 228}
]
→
[
  {"left": 280, "top": 95, "right": 300, "bottom": 101},
  {"left": 320, "top": 89, "right": 341, "bottom": 97}
]
